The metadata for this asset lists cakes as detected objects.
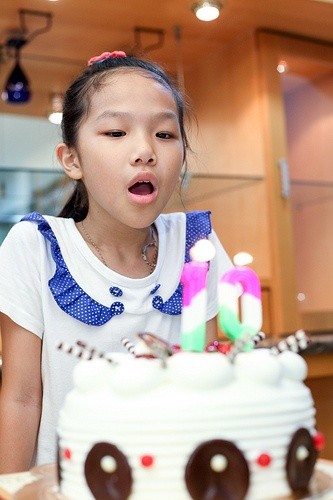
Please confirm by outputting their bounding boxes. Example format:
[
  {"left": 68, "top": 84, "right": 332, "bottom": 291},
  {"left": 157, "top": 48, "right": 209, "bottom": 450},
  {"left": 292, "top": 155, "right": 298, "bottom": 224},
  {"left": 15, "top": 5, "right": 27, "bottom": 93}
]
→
[{"left": 58, "top": 348, "right": 316, "bottom": 500}]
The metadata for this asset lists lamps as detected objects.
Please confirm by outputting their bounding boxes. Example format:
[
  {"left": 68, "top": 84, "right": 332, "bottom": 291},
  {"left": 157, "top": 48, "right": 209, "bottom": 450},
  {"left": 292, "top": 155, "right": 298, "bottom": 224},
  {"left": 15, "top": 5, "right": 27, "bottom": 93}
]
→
[
  {"left": 46, "top": 91, "right": 64, "bottom": 124},
  {"left": 191, "top": 0, "right": 222, "bottom": 22},
  {"left": 3, "top": 36, "right": 33, "bottom": 104}
]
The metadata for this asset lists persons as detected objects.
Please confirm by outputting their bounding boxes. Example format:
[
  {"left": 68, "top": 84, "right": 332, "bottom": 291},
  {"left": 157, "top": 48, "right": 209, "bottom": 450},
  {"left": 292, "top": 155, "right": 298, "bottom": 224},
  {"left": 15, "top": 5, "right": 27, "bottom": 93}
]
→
[{"left": 0, "top": 51, "right": 235, "bottom": 500}]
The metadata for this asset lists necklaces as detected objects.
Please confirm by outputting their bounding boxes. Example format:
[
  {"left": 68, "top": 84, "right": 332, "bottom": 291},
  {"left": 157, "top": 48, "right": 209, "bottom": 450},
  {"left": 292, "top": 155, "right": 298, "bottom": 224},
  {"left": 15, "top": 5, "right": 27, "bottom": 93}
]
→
[{"left": 81, "top": 215, "right": 159, "bottom": 280}]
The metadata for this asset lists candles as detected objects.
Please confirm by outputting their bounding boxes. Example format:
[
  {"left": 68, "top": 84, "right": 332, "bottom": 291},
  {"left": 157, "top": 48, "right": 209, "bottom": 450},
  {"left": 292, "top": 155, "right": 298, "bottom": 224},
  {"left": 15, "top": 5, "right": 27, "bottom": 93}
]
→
[
  {"left": 218, "top": 250, "right": 265, "bottom": 352},
  {"left": 178, "top": 237, "right": 216, "bottom": 351}
]
